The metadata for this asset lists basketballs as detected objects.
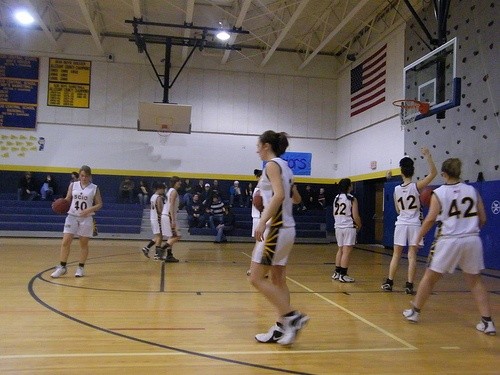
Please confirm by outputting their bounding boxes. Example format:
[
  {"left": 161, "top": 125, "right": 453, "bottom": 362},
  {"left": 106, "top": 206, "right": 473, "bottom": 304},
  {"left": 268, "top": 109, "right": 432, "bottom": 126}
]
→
[
  {"left": 421, "top": 188, "right": 433, "bottom": 208},
  {"left": 254, "top": 190, "right": 262, "bottom": 213},
  {"left": 52, "top": 198, "right": 68, "bottom": 214}
]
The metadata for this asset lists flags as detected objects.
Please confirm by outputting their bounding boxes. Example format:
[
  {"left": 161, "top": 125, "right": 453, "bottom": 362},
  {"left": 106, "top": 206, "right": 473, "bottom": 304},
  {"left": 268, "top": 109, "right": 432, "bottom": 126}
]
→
[{"left": 349, "top": 43, "right": 387, "bottom": 117}]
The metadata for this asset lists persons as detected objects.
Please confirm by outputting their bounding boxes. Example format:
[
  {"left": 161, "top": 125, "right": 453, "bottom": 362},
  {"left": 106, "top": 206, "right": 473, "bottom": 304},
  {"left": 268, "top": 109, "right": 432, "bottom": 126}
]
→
[
  {"left": 332, "top": 178, "right": 361, "bottom": 282},
  {"left": 119, "top": 177, "right": 135, "bottom": 203},
  {"left": 70, "top": 171, "right": 80, "bottom": 183},
  {"left": 381, "top": 148, "right": 436, "bottom": 294},
  {"left": 143, "top": 182, "right": 164, "bottom": 259},
  {"left": 250, "top": 130, "right": 310, "bottom": 346},
  {"left": 38, "top": 174, "right": 56, "bottom": 201},
  {"left": 157, "top": 177, "right": 180, "bottom": 262},
  {"left": 51, "top": 165, "right": 102, "bottom": 277},
  {"left": 17, "top": 171, "right": 37, "bottom": 201},
  {"left": 136, "top": 170, "right": 326, "bottom": 244},
  {"left": 402, "top": 157, "right": 496, "bottom": 336}
]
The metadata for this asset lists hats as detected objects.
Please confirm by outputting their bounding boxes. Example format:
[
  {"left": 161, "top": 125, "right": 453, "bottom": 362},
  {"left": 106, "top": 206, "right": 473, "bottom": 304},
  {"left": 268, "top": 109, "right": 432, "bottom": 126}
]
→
[
  {"left": 204, "top": 183, "right": 210, "bottom": 188},
  {"left": 234, "top": 181, "right": 239, "bottom": 184}
]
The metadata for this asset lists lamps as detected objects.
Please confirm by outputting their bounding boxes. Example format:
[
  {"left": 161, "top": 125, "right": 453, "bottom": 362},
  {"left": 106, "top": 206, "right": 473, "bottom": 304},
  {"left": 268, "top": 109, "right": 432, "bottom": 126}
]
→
[{"left": 217, "top": 7, "right": 230, "bottom": 40}]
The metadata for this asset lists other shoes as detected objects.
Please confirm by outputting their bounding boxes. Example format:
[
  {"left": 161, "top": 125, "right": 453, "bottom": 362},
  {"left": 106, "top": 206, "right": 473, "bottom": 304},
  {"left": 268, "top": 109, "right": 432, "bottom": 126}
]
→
[
  {"left": 50, "top": 265, "right": 68, "bottom": 278},
  {"left": 213, "top": 240, "right": 227, "bottom": 243},
  {"left": 382, "top": 278, "right": 393, "bottom": 291},
  {"left": 74, "top": 265, "right": 85, "bottom": 277},
  {"left": 405, "top": 282, "right": 414, "bottom": 295},
  {"left": 165, "top": 255, "right": 179, "bottom": 263},
  {"left": 339, "top": 274, "right": 355, "bottom": 283},
  {"left": 158, "top": 248, "right": 166, "bottom": 259},
  {"left": 141, "top": 246, "right": 151, "bottom": 258},
  {"left": 331, "top": 271, "right": 340, "bottom": 280},
  {"left": 153, "top": 253, "right": 160, "bottom": 259}
]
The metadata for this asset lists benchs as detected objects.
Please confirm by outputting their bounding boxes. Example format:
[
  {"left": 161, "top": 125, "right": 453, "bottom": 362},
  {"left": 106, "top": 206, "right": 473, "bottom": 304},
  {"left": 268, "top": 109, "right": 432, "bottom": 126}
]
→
[
  {"left": 0, "top": 192, "right": 144, "bottom": 239},
  {"left": 188, "top": 206, "right": 327, "bottom": 243}
]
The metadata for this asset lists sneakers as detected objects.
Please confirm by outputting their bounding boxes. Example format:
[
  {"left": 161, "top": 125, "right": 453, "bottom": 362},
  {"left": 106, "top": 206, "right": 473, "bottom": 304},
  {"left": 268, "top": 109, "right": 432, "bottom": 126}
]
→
[
  {"left": 277, "top": 311, "right": 309, "bottom": 345},
  {"left": 474, "top": 319, "right": 496, "bottom": 337},
  {"left": 402, "top": 308, "right": 420, "bottom": 323},
  {"left": 254, "top": 325, "right": 283, "bottom": 343}
]
